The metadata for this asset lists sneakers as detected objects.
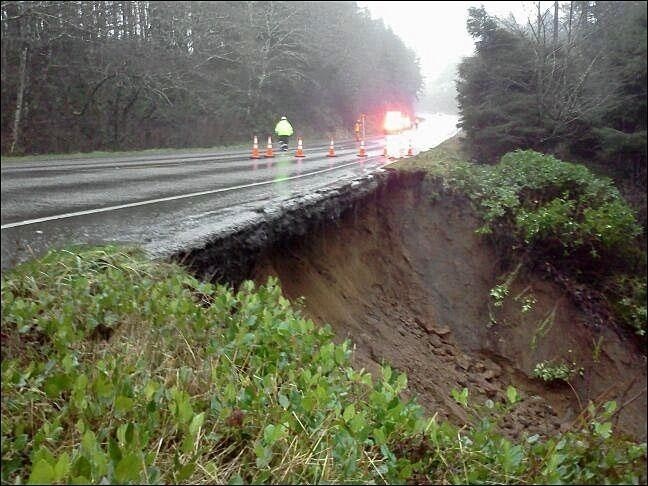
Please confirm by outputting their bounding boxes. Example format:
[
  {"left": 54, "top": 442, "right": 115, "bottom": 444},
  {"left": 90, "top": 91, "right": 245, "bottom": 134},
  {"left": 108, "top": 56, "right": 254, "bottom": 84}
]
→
[{"left": 280, "top": 145, "right": 288, "bottom": 151}]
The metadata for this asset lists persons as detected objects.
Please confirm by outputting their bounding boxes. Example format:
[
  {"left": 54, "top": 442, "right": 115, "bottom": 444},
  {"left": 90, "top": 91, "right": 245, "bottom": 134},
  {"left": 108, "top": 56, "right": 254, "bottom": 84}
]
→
[
  {"left": 354, "top": 119, "right": 363, "bottom": 141},
  {"left": 275, "top": 117, "right": 293, "bottom": 151}
]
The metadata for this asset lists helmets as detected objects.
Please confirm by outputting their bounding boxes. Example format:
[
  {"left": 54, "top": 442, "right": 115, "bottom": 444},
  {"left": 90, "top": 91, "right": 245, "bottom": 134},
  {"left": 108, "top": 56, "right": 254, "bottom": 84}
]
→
[{"left": 281, "top": 116, "right": 287, "bottom": 120}]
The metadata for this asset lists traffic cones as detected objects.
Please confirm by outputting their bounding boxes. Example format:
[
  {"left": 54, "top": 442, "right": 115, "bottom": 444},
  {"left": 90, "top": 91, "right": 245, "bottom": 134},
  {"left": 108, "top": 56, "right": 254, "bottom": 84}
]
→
[
  {"left": 251, "top": 136, "right": 260, "bottom": 159},
  {"left": 405, "top": 138, "right": 414, "bottom": 156},
  {"left": 265, "top": 134, "right": 274, "bottom": 157},
  {"left": 294, "top": 136, "right": 306, "bottom": 157},
  {"left": 326, "top": 135, "right": 336, "bottom": 157},
  {"left": 356, "top": 137, "right": 368, "bottom": 157},
  {"left": 381, "top": 138, "right": 389, "bottom": 156}
]
